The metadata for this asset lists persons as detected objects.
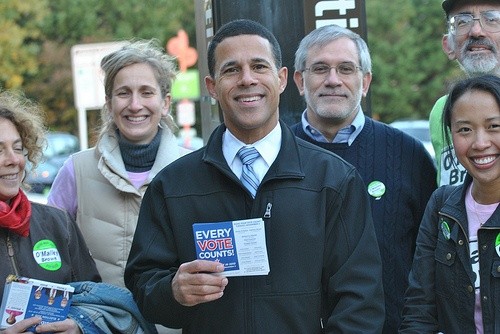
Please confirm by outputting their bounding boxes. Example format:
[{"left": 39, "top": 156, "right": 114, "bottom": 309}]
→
[
  {"left": 5, "top": 309, "right": 23, "bottom": 325},
  {"left": 33, "top": 285, "right": 73, "bottom": 308},
  {"left": 124, "top": 19, "right": 385, "bottom": 334},
  {"left": 46, "top": 37, "right": 194, "bottom": 289},
  {"left": 429, "top": 0, "right": 500, "bottom": 188},
  {"left": 398, "top": 74, "right": 500, "bottom": 334},
  {"left": 0, "top": 89, "right": 103, "bottom": 334},
  {"left": 290, "top": 24, "right": 437, "bottom": 334}
]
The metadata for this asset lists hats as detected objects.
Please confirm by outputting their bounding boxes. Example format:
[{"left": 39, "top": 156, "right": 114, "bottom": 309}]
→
[{"left": 442, "top": 0, "right": 453, "bottom": 13}]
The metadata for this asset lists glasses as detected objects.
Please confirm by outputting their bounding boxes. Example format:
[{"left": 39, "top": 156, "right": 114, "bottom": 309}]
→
[
  {"left": 448, "top": 11, "right": 500, "bottom": 35},
  {"left": 301, "top": 63, "right": 361, "bottom": 78}
]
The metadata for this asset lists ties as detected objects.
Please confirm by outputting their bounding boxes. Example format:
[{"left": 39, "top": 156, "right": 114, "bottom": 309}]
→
[{"left": 236, "top": 146, "right": 260, "bottom": 200}]
